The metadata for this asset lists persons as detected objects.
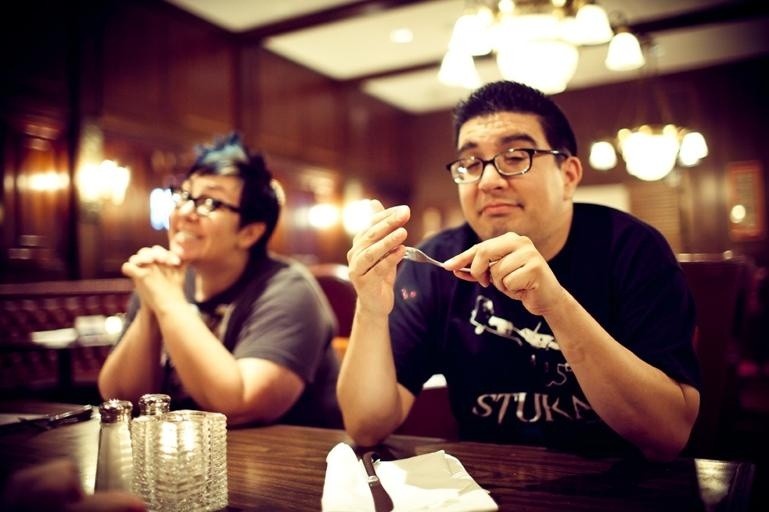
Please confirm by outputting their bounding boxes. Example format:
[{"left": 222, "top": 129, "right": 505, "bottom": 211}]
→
[
  {"left": 332, "top": 76, "right": 705, "bottom": 469},
  {"left": 95, "top": 129, "right": 348, "bottom": 432}
]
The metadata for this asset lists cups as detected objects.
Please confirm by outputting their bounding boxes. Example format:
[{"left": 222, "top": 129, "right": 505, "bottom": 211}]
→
[{"left": 131, "top": 410, "right": 227, "bottom": 510}]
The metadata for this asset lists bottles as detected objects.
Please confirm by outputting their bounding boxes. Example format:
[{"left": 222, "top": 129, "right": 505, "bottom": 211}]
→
[{"left": 94, "top": 390, "right": 172, "bottom": 495}]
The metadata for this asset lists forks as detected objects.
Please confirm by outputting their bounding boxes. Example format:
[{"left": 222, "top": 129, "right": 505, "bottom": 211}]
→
[{"left": 402, "top": 246, "right": 498, "bottom": 272}]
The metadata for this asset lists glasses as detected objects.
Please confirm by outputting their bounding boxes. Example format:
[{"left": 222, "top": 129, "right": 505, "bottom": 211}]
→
[
  {"left": 168, "top": 184, "right": 241, "bottom": 216},
  {"left": 445, "top": 146, "right": 569, "bottom": 184}
]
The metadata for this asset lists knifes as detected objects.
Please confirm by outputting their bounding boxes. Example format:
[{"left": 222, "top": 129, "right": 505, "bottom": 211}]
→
[{"left": 352, "top": 449, "right": 393, "bottom": 512}]
